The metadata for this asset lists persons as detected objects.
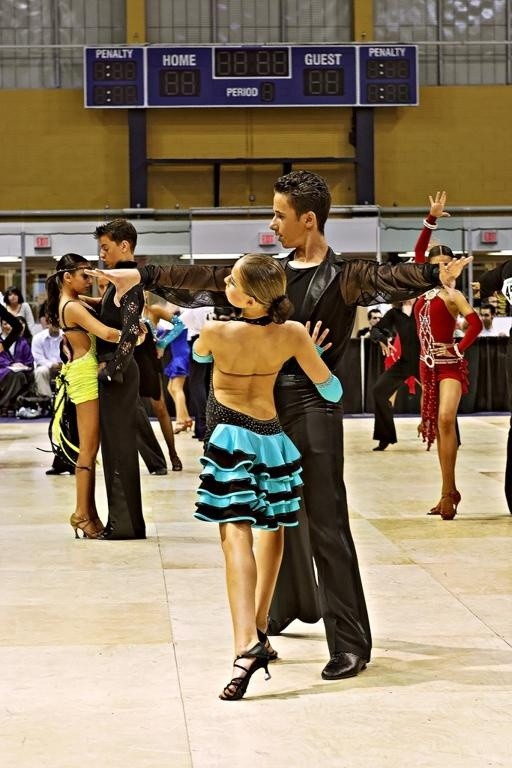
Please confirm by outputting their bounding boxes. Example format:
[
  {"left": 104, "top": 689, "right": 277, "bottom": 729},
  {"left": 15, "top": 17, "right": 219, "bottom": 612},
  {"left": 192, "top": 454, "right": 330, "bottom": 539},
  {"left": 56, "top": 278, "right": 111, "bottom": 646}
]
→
[
  {"left": 82, "top": 167, "right": 474, "bottom": 680},
  {"left": 480, "top": 254, "right": 512, "bottom": 518},
  {"left": 72, "top": 215, "right": 147, "bottom": 539},
  {"left": 190, "top": 252, "right": 346, "bottom": 701},
  {"left": 0, "top": 232, "right": 512, "bottom": 485},
  {"left": 44, "top": 251, "right": 149, "bottom": 538},
  {"left": 413, "top": 189, "right": 486, "bottom": 521}
]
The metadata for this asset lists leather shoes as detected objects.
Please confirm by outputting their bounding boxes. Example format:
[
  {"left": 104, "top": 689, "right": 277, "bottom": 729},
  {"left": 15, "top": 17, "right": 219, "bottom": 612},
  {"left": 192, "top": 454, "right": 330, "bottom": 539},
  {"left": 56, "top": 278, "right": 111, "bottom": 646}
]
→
[
  {"left": 322, "top": 652, "right": 366, "bottom": 680},
  {"left": 155, "top": 467, "right": 167, "bottom": 474},
  {"left": 105, "top": 523, "right": 131, "bottom": 539},
  {"left": 192, "top": 434, "right": 206, "bottom": 440},
  {"left": 372, "top": 438, "right": 397, "bottom": 451},
  {"left": 46, "top": 467, "right": 75, "bottom": 475}
]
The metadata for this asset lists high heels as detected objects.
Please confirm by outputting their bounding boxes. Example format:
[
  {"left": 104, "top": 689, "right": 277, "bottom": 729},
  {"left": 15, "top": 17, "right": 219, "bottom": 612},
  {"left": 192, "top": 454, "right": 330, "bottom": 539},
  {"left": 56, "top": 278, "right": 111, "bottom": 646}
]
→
[
  {"left": 170, "top": 456, "right": 182, "bottom": 471},
  {"left": 219, "top": 627, "right": 278, "bottom": 700},
  {"left": 173, "top": 419, "right": 193, "bottom": 433},
  {"left": 427, "top": 490, "right": 460, "bottom": 520},
  {"left": 71, "top": 511, "right": 105, "bottom": 539}
]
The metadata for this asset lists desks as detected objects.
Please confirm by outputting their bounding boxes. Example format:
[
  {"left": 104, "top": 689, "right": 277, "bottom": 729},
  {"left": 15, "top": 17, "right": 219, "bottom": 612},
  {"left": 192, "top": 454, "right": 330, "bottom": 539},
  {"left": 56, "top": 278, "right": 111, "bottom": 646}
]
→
[
  {"left": 143, "top": 334, "right": 363, "bottom": 418},
  {"left": 363, "top": 335, "right": 512, "bottom": 415}
]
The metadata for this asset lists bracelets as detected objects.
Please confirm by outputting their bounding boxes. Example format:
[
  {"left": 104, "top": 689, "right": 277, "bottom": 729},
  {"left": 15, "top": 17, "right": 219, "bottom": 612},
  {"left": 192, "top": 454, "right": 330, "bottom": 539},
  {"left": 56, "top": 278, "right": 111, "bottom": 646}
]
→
[{"left": 423, "top": 218, "right": 439, "bottom": 230}]
